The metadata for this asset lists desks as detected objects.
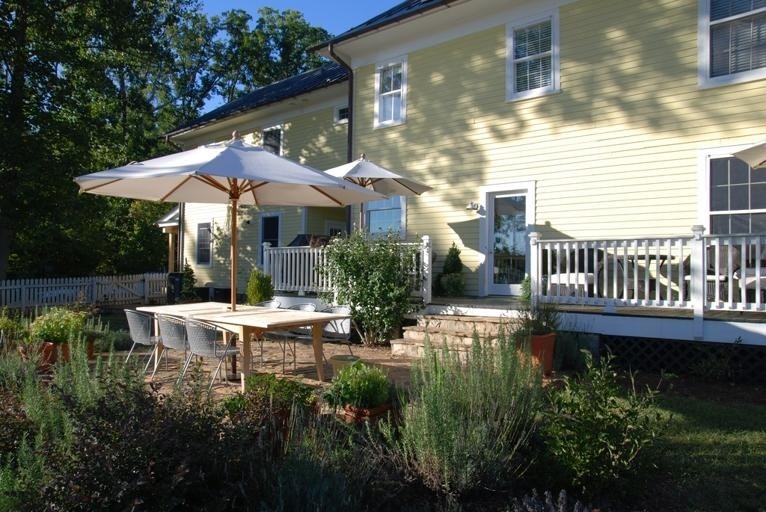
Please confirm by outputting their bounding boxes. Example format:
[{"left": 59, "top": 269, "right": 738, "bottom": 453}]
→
[{"left": 608, "top": 254, "right": 675, "bottom": 300}]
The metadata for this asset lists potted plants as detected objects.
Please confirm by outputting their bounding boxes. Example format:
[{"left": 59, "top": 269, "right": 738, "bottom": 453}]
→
[
  {"left": 37, "top": 306, "right": 94, "bottom": 362},
  {"left": 176, "top": 266, "right": 202, "bottom": 304},
  {"left": 225, "top": 373, "right": 313, "bottom": 437},
  {"left": 17, "top": 336, "right": 54, "bottom": 369},
  {"left": 504, "top": 298, "right": 571, "bottom": 378},
  {"left": 318, "top": 359, "right": 399, "bottom": 427}
]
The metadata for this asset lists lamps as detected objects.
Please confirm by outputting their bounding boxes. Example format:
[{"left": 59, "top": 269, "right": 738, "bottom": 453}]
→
[{"left": 466, "top": 202, "right": 478, "bottom": 210}]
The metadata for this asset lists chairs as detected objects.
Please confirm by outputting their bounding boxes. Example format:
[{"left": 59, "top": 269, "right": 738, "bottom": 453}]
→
[
  {"left": 682, "top": 245, "right": 741, "bottom": 301},
  {"left": 122, "top": 302, "right": 355, "bottom": 400},
  {"left": 733, "top": 246, "right": 766, "bottom": 279}
]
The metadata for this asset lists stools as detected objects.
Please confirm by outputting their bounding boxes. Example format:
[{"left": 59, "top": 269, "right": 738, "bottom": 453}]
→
[
  {"left": 547, "top": 274, "right": 594, "bottom": 297},
  {"left": 736, "top": 275, "right": 766, "bottom": 315}
]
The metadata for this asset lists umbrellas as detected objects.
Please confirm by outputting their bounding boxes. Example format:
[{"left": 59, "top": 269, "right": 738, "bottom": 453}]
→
[
  {"left": 319, "top": 152, "right": 433, "bottom": 234},
  {"left": 70, "top": 132, "right": 386, "bottom": 380}
]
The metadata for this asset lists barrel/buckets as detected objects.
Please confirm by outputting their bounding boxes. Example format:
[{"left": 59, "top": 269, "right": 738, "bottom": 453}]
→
[{"left": 328, "top": 355, "right": 360, "bottom": 378}]
